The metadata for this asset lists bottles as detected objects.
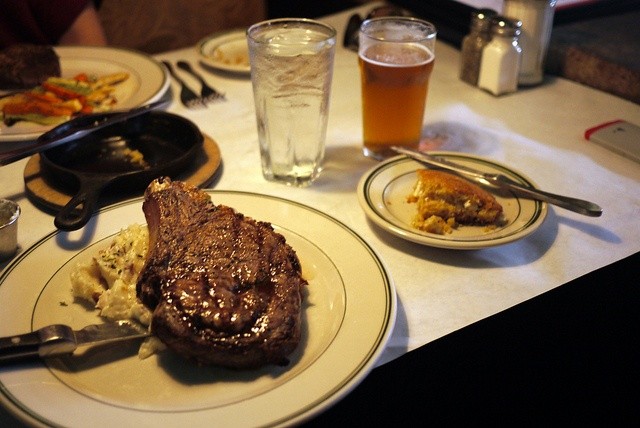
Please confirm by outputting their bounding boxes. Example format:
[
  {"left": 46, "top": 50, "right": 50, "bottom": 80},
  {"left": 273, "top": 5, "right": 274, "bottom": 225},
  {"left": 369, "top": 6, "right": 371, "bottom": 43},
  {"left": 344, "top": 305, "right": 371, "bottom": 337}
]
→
[
  {"left": 475, "top": 15, "right": 523, "bottom": 98},
  {"left": 458, "top": 7, "right": 499, "bottom": 88},
  {"left": 497, "top": 0, "right": 557, "bottom": 88}
]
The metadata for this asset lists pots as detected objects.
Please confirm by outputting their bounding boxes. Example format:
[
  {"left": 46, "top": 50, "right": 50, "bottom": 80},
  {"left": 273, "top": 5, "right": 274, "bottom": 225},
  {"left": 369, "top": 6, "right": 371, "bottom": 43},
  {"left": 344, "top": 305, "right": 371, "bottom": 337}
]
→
[{"left": 37, "top": 110, "right": 204, "bottom": 231}]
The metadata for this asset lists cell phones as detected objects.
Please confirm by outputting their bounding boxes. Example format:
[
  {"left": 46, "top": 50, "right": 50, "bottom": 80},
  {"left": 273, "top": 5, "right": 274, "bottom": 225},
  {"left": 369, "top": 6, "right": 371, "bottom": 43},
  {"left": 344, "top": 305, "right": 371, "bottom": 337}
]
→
[{"left": 585, "top": 119, "right": 636, "bottom": 165}]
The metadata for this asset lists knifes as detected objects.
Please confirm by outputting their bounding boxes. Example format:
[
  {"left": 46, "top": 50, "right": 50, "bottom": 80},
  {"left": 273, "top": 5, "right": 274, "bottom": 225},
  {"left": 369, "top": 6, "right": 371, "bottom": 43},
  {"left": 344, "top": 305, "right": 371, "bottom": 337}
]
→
[
  {"left": 0, "top": 321, "right": 150, "bottom": 362},
  {"left": 389, "top": 144, "right": 604, "bottom": 218},
  {"left": 0, "top": 100, "right": 169, "bottom": 169}
]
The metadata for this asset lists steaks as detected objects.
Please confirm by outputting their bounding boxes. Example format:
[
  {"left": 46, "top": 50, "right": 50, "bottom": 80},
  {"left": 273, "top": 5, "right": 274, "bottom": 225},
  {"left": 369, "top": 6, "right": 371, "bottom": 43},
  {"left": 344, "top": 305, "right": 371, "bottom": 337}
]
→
[{"left": 135, "top": 176, "right": 303, "bottom": 364}]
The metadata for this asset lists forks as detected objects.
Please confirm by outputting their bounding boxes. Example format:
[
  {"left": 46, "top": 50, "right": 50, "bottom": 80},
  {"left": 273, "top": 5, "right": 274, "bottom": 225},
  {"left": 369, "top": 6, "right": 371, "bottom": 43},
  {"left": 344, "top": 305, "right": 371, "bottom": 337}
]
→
[
  {"left": 161, "top": 60, "right": 208, "bottom": 111},
  {"left": 177, "top": 61, "right": 226, "bottom": 102}
]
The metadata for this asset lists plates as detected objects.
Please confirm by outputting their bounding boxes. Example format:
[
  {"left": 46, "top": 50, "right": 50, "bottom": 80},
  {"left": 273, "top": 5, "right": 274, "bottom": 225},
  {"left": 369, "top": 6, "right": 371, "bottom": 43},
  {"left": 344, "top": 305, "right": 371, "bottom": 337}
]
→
[
  {"left": 195, "top": 27, "right": 332, "bottom": 76},
  {"left": 0, "top": 189, "right": 399, "bottom": 428},
  {"left": 356, "top": 150, "right": 548, "bottom": 251},
  {"left": 0, "top": 44, "right": 172, "bottom": 147}
]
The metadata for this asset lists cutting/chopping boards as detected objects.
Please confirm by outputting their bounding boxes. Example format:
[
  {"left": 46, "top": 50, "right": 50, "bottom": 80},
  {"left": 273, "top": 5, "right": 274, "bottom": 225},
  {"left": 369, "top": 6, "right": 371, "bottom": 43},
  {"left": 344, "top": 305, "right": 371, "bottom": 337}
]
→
[{"left": 24, "top": 131, "right": 222, "bottom": 217}]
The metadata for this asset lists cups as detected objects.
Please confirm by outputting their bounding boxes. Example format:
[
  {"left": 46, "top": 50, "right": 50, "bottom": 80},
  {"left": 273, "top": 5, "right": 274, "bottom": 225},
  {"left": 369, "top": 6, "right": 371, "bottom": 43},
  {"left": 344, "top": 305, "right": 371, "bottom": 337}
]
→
[
  {"left": 0, "top": 198, "right": 22, "bottom": 264},
  {"left": 245, "top": 17, "right": 337, "bottom": 187},
  {"left": 357, "top": 15, "right": 438, "bottom": 162}
]
